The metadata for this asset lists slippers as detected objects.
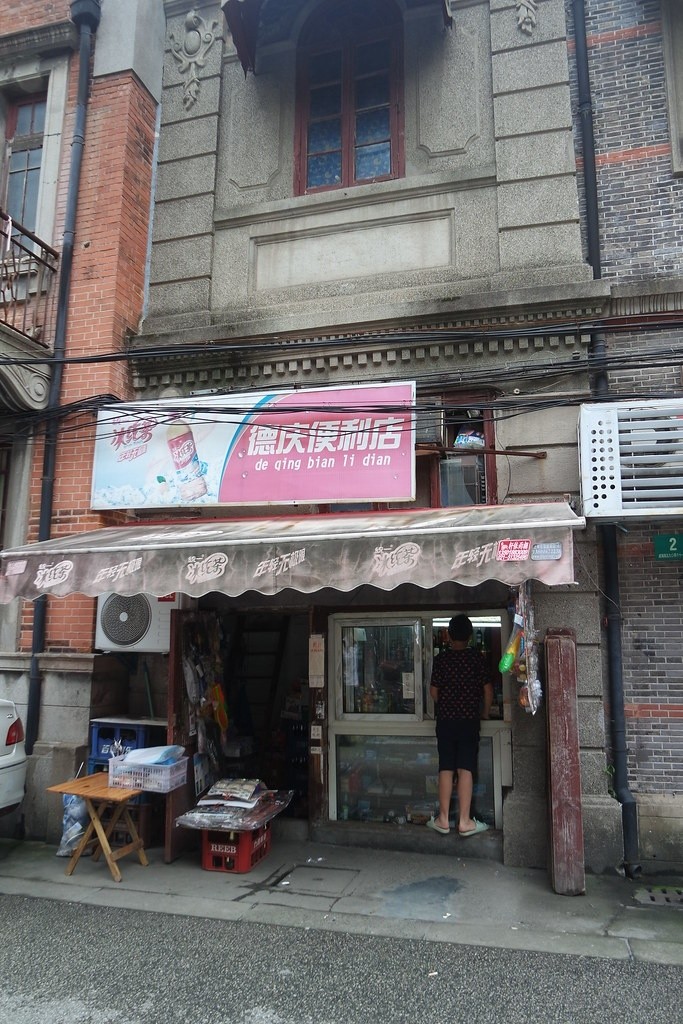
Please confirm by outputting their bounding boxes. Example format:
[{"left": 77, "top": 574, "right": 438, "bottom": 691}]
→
[
  {"left": 426, "top": 816, "right": 450, "bottom": 834},
  {"left": 458, "top": 817, "right": 490, "bottom": 836}
]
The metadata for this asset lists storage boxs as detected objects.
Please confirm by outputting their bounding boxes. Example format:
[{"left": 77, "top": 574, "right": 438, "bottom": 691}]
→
[
  {"left": 87, "top": 722, "right": 167, "bottom": 848},
  {"left": 201, "top": 817, "right": 273, "bottom": 873},
  {"left": 108, "top": 755, "right": 189, "bottom": 794}
]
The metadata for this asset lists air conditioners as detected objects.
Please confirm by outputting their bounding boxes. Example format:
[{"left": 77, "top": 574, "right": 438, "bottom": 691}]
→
[
  {"left": 604, "top": 402, "right": 683, "bottom": 469},
  {"left": 94, "top": 591, "right": 199, "bottom": 654}
]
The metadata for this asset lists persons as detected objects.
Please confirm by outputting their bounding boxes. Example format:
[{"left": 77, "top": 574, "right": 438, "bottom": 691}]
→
[{"left": 424, "top": 613, "right": 492, "bottom": 837}]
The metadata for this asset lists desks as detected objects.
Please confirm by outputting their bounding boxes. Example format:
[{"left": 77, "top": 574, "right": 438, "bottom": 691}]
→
[{"left": 46, "top": 772, "right": 148, "bottom": 882}]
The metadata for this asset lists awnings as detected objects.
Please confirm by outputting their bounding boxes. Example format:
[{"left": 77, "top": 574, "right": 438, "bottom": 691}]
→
[{"left": 0, "top": 499, "right": 585, "bottom": 604}]
